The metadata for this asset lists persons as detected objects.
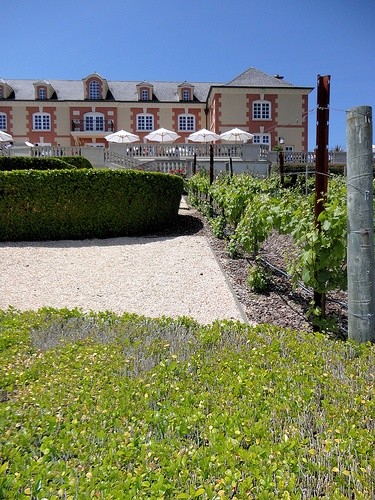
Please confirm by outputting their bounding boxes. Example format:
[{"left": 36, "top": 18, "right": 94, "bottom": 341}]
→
[
  {"left": 175, "top": 147, "right": 179, "bottom": 157},
  {"left": 56, "top": 144, "right": 63, "bottom": 156},
  {"left": 7, "top": 141, "right": 13, "bottom": 149},
  {"left": 128, "top": 145, "right": 154, "bottom": 157},
  {"left": 34, "top": 144, "right": 41, "bottom": 157}
]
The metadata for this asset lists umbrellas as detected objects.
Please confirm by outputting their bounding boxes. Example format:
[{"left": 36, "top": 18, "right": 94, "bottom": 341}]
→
[
  {"left": 220, "top": 128, "right": 254, "bottom": 145},
  {"left": 104, "top": 129, "right": 141, "bottom": 144},
  {"left": 0, "top": 131, "right": 12, "bottom": 142},
  {"left": 188, "top": 128, "right": 220, "bottom": 144},
  {"left": 145, "top": 128, "right": 181, "bottom": 143}
]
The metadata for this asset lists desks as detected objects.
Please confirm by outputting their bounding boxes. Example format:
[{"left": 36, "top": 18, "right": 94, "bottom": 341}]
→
[
  {"left": 194, "top": 153, "right": 243, "bottom": 157},
  {"left": 132, "top": 153, "right": 178, "bottom": 157}
]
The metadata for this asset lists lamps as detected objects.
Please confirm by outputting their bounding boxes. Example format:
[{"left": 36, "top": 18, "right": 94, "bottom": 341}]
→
[{"left": 279, "top": 135, "right": 285, "bottom": 145}]
